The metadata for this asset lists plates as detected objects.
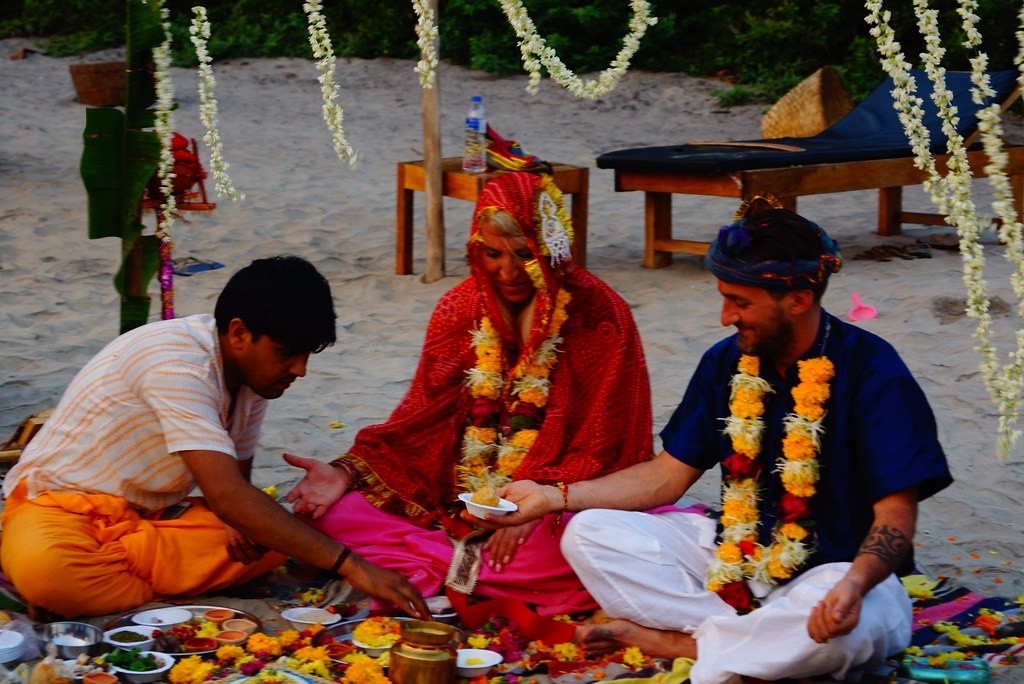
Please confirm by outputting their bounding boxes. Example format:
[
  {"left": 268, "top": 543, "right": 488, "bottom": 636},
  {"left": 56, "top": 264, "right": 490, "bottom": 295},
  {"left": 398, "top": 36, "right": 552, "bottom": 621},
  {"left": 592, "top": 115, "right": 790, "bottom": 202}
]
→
[{"left": 0, "top": 629, "right": 26, "bottom": 664}]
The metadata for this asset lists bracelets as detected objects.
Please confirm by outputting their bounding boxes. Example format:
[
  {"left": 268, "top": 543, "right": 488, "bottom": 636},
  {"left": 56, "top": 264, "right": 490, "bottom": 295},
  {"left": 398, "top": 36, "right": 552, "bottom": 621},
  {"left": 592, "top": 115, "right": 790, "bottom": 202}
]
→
[
  {"left": 554, "top": 482, "right": 568, "bottom": 511},
  {"left": 330, "top": 548, "right": 352, "bottom": 574}
]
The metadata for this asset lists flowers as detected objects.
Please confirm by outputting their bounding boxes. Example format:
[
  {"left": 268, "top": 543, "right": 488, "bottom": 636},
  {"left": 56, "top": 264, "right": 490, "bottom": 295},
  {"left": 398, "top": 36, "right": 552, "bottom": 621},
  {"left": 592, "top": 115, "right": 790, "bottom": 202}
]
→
[
  {"left": 411, "top": 0, "right": 439, "bottom": 91},
  {"left": 187, "top": 4, "right": 245, "bottom": 204},
  {"left": 863, "top": 0, "right": 1024, "bottom": 465},
  {"left": 302, "top": 0, "right": 359, "bottom": 169},
  {"left": 28, "top": 614, "right": 659, "bottom": 684},
  {"left": 499, "top": 0, "right": 658, "bottom": 101},
  {"left": 141, "top": 0, "right": 178, "bottom": 243}
]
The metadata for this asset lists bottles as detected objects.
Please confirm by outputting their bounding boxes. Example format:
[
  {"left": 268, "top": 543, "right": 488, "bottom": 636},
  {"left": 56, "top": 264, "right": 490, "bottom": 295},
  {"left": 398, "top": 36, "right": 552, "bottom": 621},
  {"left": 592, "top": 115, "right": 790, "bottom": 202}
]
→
[
  {"left": 388, "top": 621, "right": 457, "bottom": 684},
  {"left": 462, "top": 96, "right": 488, "bottom": 173}
]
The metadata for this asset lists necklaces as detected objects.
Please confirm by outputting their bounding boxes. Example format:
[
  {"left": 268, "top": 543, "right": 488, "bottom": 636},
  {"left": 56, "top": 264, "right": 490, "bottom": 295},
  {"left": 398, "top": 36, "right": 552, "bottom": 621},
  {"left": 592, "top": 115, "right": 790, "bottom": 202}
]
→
[
  {"left": 459, "top": 283, "right": 574, "bottom": 496},
  {"left": 706, "top": 341, "right": 834, "bottom": 617}
]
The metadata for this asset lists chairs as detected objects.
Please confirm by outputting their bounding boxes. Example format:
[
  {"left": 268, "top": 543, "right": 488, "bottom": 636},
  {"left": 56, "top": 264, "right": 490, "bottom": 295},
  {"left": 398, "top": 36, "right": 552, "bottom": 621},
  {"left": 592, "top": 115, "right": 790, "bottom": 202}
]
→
[{"left": 596, "top": 68, "right": 1024, "bottom": 273}]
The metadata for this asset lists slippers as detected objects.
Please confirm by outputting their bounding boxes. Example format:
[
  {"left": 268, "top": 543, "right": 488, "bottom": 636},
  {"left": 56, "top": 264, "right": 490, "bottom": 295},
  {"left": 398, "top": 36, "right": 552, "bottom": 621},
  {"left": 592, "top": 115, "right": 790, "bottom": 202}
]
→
[{"left": 855, "top": 233, "right": 960, "bottom": 263}]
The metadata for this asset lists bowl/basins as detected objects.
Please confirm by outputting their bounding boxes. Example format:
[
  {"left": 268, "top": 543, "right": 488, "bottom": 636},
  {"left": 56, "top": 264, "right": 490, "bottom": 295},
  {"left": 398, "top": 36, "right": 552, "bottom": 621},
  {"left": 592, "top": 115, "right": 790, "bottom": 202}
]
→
[
  {"left": 351, "top": 639, "right": 391, "bottom": 657},
  {"left": 109, "top": 651, "right": 176, "bottom": 684},
  {"left": 215, "top": 630, "right": 249, "bottom": 646},
  {"left": 33, "top": 621, "right": 104, "bottom": 660},
  {"left": 102, "top": 625, "right": 163, "bottom": 651},
  {"left": 204, "top": 608, "right": 235, "bottom": 628},
  {"left": 457, "top": 648, "right": 504, "bottom": 677},
  {"left": 222, "top": 619, "right": 258, "bottom": 635},
  {"left": 281, "top": 607, "right": 341, "bottom": 632},
  {"left": 186, "top": 638, "right": 218, "bottom": 652},
  {"left": 425, "top": 596, "right": 458, "bottom": 623},
  {"left": 132, "top": 608, "right": 192, "bottom": 632},
  {"left": 458, "top": 493, "right": 517, "bottom": 519}
]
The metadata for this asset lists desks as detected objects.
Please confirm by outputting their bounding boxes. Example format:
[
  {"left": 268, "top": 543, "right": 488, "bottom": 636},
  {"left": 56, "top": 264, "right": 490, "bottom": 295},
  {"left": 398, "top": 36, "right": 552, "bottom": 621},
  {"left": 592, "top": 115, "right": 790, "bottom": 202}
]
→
[{"left": 395, "top": 155, "right": 590, "bottom": 276}]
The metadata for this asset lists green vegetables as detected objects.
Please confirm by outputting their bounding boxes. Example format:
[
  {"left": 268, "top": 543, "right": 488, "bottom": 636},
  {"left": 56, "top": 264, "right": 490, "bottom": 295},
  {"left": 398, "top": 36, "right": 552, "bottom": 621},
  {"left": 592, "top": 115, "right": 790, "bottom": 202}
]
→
[{"left": 108, "top": 649, "right": 159, "bottom": 670}]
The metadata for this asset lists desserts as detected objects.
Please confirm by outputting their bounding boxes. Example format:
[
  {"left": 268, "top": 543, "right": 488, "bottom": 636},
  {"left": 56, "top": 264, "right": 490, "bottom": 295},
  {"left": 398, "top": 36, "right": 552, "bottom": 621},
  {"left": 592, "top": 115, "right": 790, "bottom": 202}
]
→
[{"left": 470, "top": 487, "right": 501, "bottom": 507}]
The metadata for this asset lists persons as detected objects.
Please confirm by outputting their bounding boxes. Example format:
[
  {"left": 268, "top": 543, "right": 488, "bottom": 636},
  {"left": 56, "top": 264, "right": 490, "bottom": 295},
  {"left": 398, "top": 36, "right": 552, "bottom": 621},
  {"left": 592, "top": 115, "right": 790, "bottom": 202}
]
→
[
  {"left": 0, "top": 253, "right": 434, "bottom": 628},
  {"left": 281, "top": 170, "right": 655, "bottom": 603},
  {"left": 459, "top": 207, "right": 955, "bottom": 684}
]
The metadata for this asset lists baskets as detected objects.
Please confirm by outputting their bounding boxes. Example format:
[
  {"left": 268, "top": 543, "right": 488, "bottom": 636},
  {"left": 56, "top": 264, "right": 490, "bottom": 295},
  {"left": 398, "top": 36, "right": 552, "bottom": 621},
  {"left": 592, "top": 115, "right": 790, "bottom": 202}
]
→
[
  {"left": 761, "top": 65, "right": 850, "bottom": 138},
  {"left": 69, "top": 60, "right": 126, "bottom": 108}
]
